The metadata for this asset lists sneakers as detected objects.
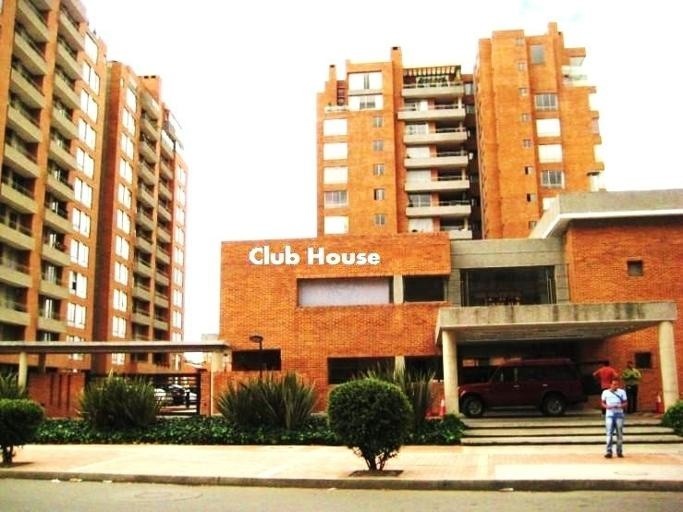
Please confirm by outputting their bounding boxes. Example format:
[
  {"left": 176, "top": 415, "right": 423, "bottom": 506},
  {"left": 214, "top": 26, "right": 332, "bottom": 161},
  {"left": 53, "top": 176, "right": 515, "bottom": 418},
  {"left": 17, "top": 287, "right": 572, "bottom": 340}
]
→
[
  {"left": 605, "top": 453, "right": 612, "bottom": 458},
  {"left": 618, "top": 454, "right": 623, "bottom": 457}
]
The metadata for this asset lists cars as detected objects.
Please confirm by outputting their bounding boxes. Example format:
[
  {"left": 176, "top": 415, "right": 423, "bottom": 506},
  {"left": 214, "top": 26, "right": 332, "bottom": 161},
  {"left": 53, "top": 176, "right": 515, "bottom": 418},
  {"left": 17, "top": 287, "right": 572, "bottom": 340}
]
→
[{"left": 151, "top": 385, "right": 198, "bottom": 407}]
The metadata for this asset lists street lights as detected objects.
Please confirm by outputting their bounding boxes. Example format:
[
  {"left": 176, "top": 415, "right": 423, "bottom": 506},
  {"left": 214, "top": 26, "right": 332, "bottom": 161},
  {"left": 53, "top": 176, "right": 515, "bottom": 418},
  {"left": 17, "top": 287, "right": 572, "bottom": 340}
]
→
[{"left": 251, "top": 334, "right": 265, "bottom": 380}]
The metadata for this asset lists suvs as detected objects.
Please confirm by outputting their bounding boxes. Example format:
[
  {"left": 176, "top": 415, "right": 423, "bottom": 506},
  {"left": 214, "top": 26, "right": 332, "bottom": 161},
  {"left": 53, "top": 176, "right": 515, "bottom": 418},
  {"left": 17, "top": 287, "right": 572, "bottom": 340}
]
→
[{"left": 457, "top": 358, "right": 587, "bottom": 418}]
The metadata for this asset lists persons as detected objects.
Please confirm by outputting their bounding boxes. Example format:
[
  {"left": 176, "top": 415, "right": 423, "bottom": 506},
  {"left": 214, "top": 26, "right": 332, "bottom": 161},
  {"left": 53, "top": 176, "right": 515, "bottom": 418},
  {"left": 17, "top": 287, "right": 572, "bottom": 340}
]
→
[
  {"left": 593, "top": 358, "right": 620, "bottom": 418},
  {"left": 600, "top": 376, "right": 629, "bottom": 459},
  {"left": 622, "top": 360, "right": 642, "bottom": 415},
  {"left": 593, "top": 360, "right": 603, "bottom": 395}
]
